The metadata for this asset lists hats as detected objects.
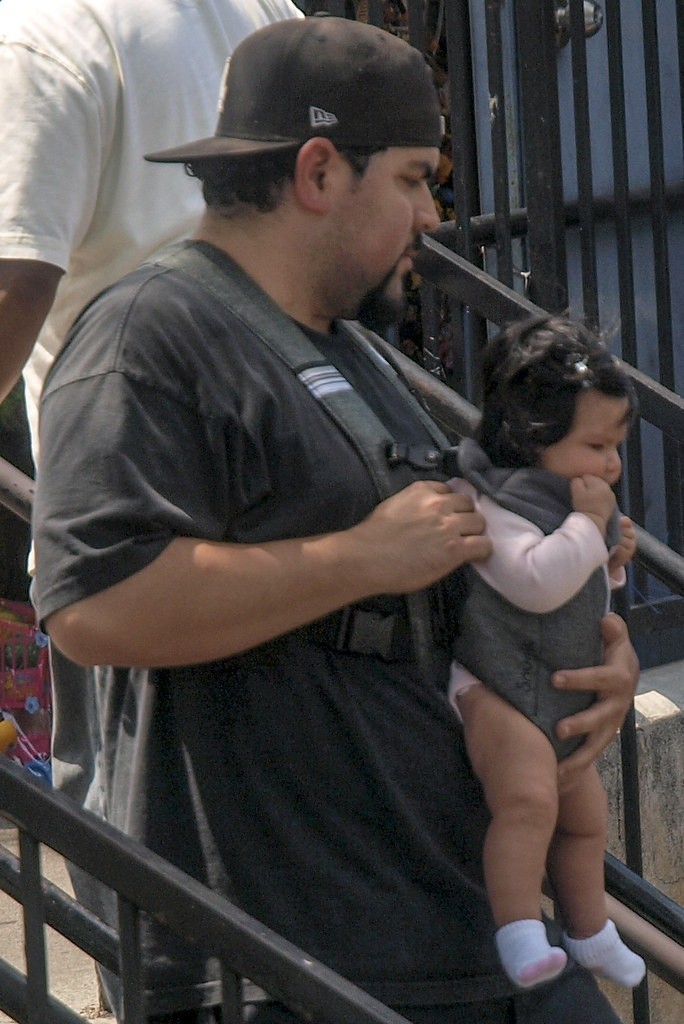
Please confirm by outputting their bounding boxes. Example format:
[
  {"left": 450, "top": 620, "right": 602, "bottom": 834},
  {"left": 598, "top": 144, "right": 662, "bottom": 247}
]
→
[{"left": 138, "top": 14, "right": 444, "bottom": 161}]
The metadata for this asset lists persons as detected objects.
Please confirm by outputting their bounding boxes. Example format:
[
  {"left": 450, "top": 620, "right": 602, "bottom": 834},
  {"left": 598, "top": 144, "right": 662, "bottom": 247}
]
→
[
  {"left": 1, "top": 1, "right": 322, "bottom": 1024},
  {"left": 31, "top": 16, "right": 645, "bottom": 1024},
  {"left": 443, "top": 310, "right": 653, "bottom": 993}
]
